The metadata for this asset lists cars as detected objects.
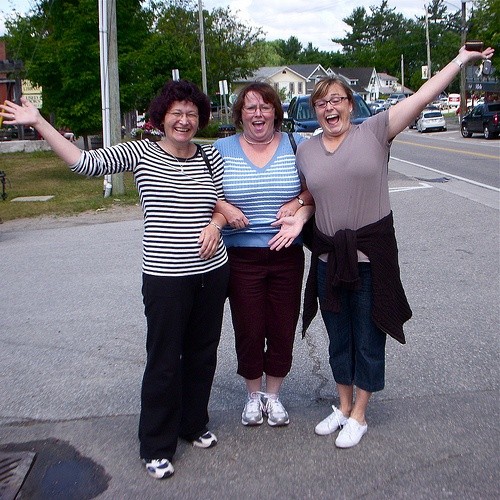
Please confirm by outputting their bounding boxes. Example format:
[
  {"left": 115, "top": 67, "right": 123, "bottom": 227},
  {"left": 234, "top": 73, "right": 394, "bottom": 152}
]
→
[
  {"left": 459, "top": 102, "right": 500, "bottom": 140},
  {"left": 408, "top": 112, "right": 423, "bottom": 129},
  {"left": 375, "top": 99, "right": 391, "bottom": 109},
  {"left": 375, "top": 107, "right": 387, "bottom": 114},
  {"left": 415, "top": 110, "right": 448, "bottom": 133},
  {"left": 431, "top": 101, "right": 448, "bottom": 110}
]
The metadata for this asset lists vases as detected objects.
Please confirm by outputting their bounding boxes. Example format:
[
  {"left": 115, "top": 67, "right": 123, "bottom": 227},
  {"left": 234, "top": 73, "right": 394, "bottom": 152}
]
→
[{"left": 135, "top": 133, "right": 162, "bottom": 142}]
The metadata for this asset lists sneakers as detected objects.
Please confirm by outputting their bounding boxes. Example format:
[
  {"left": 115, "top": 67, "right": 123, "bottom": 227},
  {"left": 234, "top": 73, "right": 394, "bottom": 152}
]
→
[
  {"left": 241, "top": 391, "right": 266, "bottom": 426},
  {"left": 141, "top": 456, "right": 174, "bottom": 479},
  {"left": 335, "top": 418, "right": 367, "bottom": 448},
  {"left": 263, "top": 392, "right": 289, "bottom": 426},
  {"left": 191, "top": 431, "right": 216, "bottom": 449},
  {"left": 315, "top": 404, "right": 348, "bottom": 436}
]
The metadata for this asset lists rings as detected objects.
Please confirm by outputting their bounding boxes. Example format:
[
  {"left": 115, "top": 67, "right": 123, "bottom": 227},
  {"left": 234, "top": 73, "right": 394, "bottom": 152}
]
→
[{"left": 13, "top": 113, "right": 15, "bottom": 119}]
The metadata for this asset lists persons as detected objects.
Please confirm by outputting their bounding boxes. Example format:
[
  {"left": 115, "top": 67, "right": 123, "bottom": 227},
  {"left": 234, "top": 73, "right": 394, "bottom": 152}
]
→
[
  {"left": 213, "top": 83, "right": 315, "bottom": 427},
  {"left": 0, "top": 80, "right": 228, "bottom": 479},
  {"left": 269, "top": 45, "right": 494, "bottom": 448}
]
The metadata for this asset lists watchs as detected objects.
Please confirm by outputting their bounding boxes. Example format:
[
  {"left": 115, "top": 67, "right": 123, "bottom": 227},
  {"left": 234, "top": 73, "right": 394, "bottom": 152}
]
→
[{"left": 296, "top": 196, "right": 304, "bottom": 206}]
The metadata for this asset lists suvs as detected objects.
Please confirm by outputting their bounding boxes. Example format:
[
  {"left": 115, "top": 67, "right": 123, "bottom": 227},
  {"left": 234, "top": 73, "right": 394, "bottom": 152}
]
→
[
  {"left": 367, "top": 103, "right": 380, "bottom": 114},
  {"left": 281, "top": 91, "right": 375, "bottom": 139}
]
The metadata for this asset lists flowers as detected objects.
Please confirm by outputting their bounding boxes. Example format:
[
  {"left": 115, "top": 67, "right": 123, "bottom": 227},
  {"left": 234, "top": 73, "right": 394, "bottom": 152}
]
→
[{"left": 130, "top": 121, "right": 165, "bottom": 139}]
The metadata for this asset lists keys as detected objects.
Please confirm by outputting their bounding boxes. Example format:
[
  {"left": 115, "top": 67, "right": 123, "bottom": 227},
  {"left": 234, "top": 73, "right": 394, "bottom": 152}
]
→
[{"left": 477, "top": 59, "right": 491, "bottom": 81}]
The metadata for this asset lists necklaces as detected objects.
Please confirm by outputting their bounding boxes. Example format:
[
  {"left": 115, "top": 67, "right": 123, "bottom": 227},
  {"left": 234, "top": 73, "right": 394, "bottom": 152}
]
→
[
  {"left": 242, "top": 133, "right": 274, "bottom": 144},
  {"left": 163, "top": 142, "right": 190, "bottom": 172}
]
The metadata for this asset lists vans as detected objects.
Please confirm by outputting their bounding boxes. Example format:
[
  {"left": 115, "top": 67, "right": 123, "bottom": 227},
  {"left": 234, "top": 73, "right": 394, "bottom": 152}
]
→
[
  {"left": 440, "top": 97, "right": 458, "bottom": 109},
  {"left": 386, "top": 93, "right": 407, "bottom": 105}
]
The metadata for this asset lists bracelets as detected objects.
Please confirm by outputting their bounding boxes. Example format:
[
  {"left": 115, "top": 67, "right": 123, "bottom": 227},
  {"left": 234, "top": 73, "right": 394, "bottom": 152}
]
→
[
  {"left": 210, "top": 220, "right": 222, "bottom": 231},
  {"left": 452, "top": 58, "right": 465, "bottom": 69}
]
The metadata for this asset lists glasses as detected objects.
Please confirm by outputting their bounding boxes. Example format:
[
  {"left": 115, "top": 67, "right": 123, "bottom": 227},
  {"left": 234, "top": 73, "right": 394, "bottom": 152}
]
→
[
  {"left": 241, "top": 103, "right": 274, "bottom": 113},
  {"left": 166, "top": 111, "right": 198, "bottom": 120},
  {"left": 314, "top": 96, "right": 349, "bottom": 108}
]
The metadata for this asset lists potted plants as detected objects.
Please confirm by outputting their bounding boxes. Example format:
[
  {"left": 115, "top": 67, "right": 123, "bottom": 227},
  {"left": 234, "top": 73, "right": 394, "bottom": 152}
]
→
[{"left": 90, "top": 135, "right": 103, "bottom": 150}]
